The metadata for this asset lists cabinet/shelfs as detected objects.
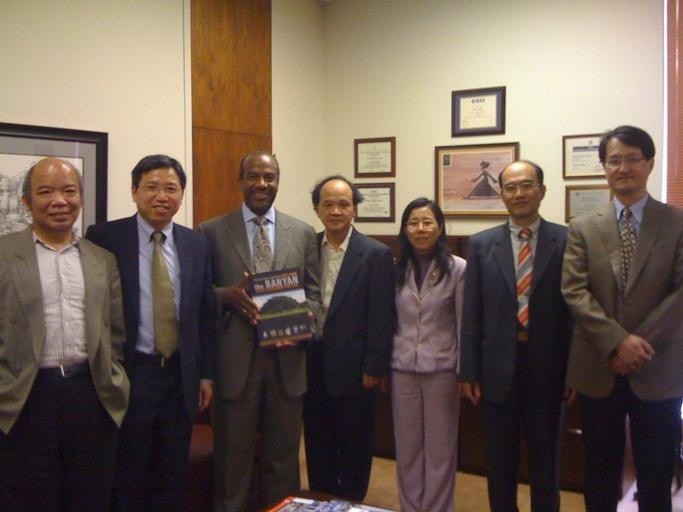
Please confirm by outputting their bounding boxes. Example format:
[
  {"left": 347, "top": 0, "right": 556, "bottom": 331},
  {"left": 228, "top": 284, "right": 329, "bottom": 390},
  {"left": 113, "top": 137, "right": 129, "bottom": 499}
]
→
[{"left": 357, "top": 233, "right": 636, "bottom": 501}]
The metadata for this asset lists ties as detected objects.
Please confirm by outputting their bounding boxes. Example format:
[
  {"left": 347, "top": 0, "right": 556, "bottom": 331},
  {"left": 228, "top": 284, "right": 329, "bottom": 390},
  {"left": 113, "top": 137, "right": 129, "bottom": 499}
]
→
[
  {"left": 621, "top": 209, "right": 637, "bottom": 288},
  {"left": 516, "top": 228, "right": 534, "bottom": 341},
  {"left": 252, "top": 218, "right": 273, "bottom": 273},
  {"left": 152, "top": 232, "right": 178, "bottom": 358}
]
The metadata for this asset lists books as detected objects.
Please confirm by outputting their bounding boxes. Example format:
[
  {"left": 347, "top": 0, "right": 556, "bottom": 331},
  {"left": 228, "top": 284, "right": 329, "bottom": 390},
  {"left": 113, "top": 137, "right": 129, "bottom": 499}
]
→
[{"left": 249, "top": 267, "right": 315, "bottom": 348}]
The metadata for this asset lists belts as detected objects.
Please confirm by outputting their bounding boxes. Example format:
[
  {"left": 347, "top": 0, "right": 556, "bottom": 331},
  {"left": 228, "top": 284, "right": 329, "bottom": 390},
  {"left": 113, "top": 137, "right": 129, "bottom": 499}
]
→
[{"left": 40, "top": 364, "right": 88, "bottom": 378}]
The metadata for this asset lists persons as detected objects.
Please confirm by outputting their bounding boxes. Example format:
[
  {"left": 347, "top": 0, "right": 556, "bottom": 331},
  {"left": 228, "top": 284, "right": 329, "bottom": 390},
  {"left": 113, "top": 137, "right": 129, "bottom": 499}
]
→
[
  {"left": 193, "top": 149, "right": 322, "bottom": 512},
  {"left": 0, "top": 157, "right": 132, "bottom": 510},
  {"left": 458, "top": 160, "right": 574, "bottom": 512},
  {"left": 379, "top": 198, "right": 468, "bottom": 512},
  {"left": 467, "top": 161, "right": 499, "bottom": 196},
  {"left": 301, "top": 175, "right": 398, "bottom": 504},
  {"left": 559, "top": 125, "right": 681, "bottom": 512},
  {"left": 84, "top": 156, "right": 215, "bottom": 512}
]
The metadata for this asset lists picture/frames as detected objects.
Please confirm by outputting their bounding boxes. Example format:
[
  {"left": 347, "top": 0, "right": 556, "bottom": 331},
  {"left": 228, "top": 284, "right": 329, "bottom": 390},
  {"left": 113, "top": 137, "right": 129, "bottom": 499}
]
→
[
  {"left": 352, "top": 181, "right": 397, "bottom": 225},
  {"left": 564, "top": 183, "right": 614, "bottom": 221},
  {"left": 431, "top": 141, "right": 520, "bottom": 220},
  {"left": 0, "top": 120, "right": 108, "bottom": 246},
  {"left": 449, "top": 84, "right": 508, "bottom": 141},
  {"left": 353, "top": 134, "right": 396, "bottom": 179},
  {"left": 560, "top": 132, "right": 612, "bottom": 179}
]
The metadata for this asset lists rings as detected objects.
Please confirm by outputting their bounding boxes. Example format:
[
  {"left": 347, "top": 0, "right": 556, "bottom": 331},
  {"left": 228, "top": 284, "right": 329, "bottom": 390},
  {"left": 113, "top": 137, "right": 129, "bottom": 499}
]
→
[{"left": 243, "top": 309, "right": 247, "bottom": 314}]
